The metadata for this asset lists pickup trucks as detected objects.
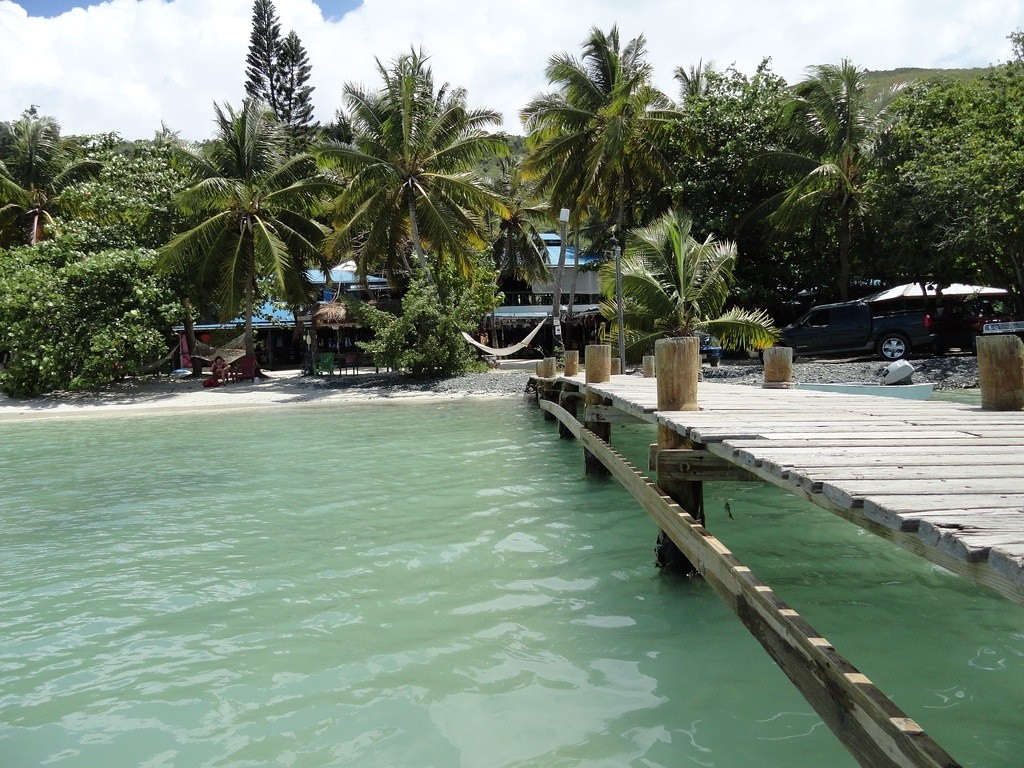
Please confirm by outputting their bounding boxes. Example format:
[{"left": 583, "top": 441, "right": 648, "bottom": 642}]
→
[{"left": 759, "top": 300, "right": 937, "bottom": 365}]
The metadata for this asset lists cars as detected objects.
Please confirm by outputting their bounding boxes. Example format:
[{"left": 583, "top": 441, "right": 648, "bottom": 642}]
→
[
  {"left": 931, "top": 298, "right": 1024, "bottom": 355},
  {"left": 700, "top": 333, "right": 724, "bottom": 367}
]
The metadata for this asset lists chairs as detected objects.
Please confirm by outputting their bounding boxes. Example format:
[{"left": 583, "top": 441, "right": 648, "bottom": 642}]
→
[
  {"left": 229, "top": 356, "right": 257, "bottom": 384},
  {"left": 208, "top": 360, "right": 234, "bottom": 384},
  {"left": 315, "top": 351, "right": 334, "bottom": 377},
  {"left": 337, "top": 353, "right": 360, "bottom": 376}
]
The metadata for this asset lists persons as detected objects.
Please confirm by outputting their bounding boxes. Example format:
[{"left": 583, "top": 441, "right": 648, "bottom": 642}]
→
[{"left": 211, "top": 355, "right": 230, "bottom": 385}]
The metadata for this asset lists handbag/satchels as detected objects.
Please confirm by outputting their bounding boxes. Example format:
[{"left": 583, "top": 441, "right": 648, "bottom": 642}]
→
[{"left": 202, "top": 377, "right": 221, "bottom": 387}]
